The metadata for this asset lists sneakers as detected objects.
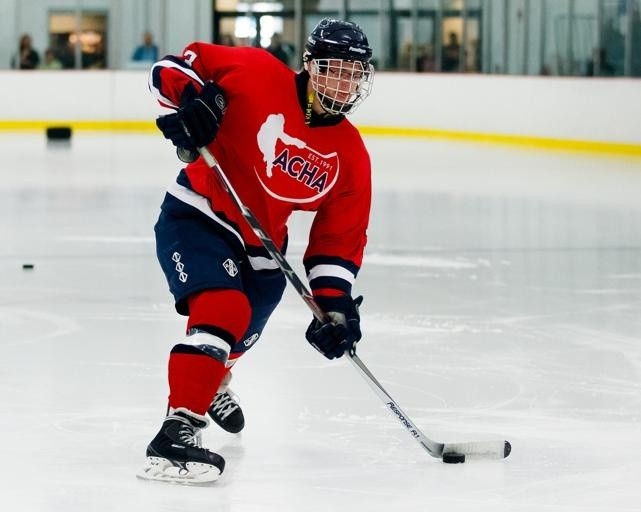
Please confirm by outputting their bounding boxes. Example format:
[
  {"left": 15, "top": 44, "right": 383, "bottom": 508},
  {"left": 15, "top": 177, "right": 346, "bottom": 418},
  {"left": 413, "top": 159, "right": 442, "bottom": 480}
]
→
[{"left": 206, "top": 389, "right": 246, "bottom": 439}]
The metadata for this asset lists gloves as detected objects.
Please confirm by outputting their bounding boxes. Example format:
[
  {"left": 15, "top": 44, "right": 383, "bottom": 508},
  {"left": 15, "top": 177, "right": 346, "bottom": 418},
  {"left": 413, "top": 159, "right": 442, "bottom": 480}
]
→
[
  {"left": 154, "top": 80, "right": 225, "bottom": 168},
  {"left": 305, "top": 288, "right": 365, "bottom": 365}
]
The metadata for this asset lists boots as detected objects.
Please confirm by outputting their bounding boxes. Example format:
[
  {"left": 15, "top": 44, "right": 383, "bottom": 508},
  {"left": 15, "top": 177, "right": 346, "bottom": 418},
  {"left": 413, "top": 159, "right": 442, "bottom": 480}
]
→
[{"left": 145, "top": 406, "right": 228, "bottom": 475}]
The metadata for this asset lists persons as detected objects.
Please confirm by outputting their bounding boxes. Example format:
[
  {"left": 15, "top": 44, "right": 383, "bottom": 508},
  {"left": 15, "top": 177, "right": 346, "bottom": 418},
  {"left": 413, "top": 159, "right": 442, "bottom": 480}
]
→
[
  {"left": 8, "top": 33, "right": 39, "bottom": 69},
  {"left": 265, "top": 32, "right": 295, "bottom": 64},
  {"left": 130, "top": 31, "right": 158, "bottom": 70},
  {"left": 441, "top": 30, "right": 467, "bottom": 72},
  {"left": 32, "top": 50, "right": 64, "bottom": 69},
  {"left": 142, "top": 16, "right": 380, "bottom": 475},
  {"left": 415, "top": 44, "right": 433, "bottom": 73}
]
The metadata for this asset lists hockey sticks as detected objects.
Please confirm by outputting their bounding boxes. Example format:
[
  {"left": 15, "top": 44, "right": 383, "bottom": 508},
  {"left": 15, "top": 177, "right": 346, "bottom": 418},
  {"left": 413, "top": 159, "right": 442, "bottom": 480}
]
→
[{"left": 182, "top": 121, "right": 510, "bottom": 460}]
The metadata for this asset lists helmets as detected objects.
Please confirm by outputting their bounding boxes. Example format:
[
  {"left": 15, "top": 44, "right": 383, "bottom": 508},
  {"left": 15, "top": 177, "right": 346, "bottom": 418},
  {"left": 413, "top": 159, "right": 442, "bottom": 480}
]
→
[{"left": 300, "top": 17, "right": 375, "bottom": 122}]
